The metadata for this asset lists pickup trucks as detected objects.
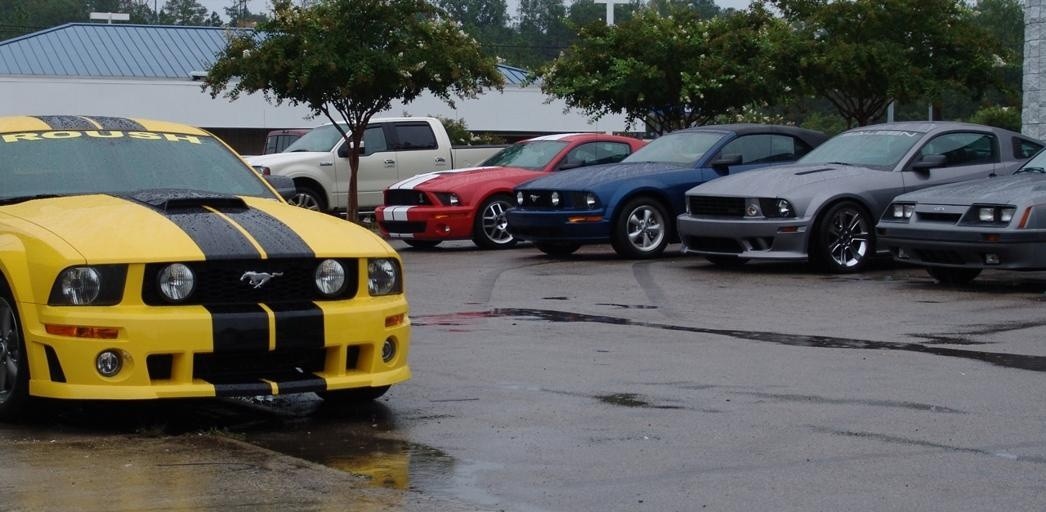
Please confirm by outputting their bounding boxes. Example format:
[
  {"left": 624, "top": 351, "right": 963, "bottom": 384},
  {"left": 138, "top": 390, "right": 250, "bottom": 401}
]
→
[{"left": 242, "top": 116, "right": 537, "bottom": 221}]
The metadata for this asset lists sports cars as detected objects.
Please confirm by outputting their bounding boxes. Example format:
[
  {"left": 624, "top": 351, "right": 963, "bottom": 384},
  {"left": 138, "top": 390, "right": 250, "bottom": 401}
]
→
[{"left": 0, "top": 115, "right": 409, "bottom": 423}]
